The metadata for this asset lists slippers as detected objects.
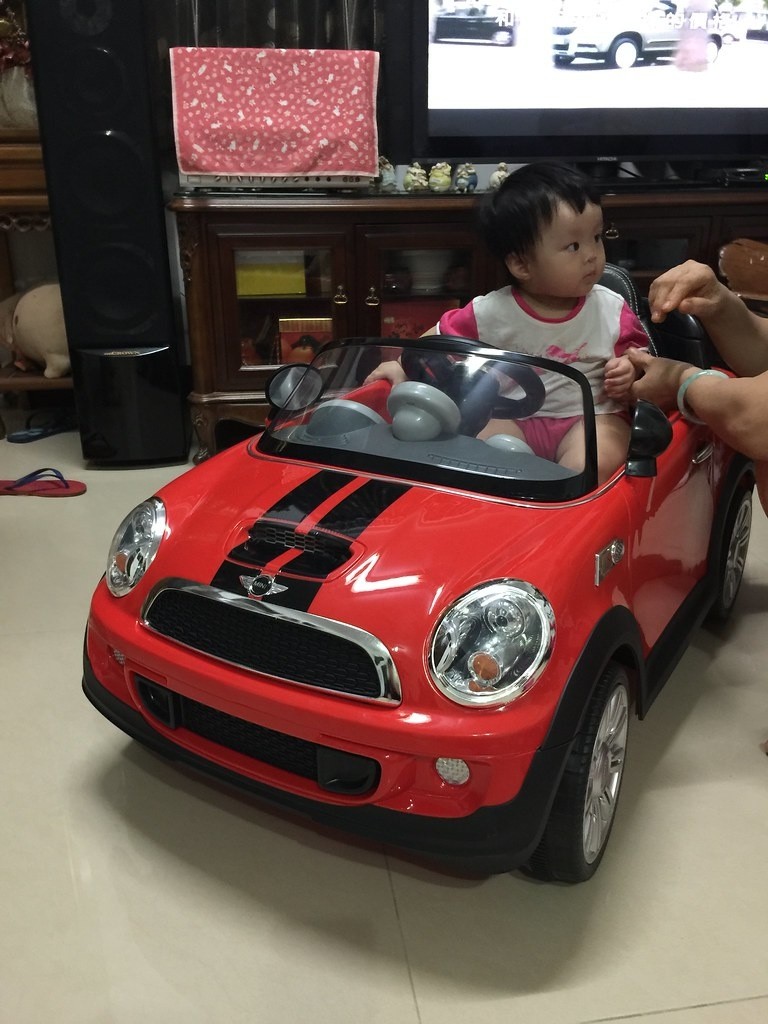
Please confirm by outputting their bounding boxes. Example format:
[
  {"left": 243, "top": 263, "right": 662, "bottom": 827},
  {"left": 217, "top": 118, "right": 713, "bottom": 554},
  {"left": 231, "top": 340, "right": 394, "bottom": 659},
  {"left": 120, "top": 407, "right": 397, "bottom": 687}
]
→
[
  {"left": 0, "top": 467, "right": 86, "bottom": 497},
  {"left": 7, "top": 409, "right": 77, "bottom": 443}
]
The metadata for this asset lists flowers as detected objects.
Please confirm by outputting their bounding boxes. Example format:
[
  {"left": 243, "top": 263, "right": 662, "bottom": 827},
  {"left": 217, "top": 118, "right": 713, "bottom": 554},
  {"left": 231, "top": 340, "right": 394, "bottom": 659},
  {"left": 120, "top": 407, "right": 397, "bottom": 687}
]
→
[{"left": 0, "top": 0, "right": 33, "bottom": 80}]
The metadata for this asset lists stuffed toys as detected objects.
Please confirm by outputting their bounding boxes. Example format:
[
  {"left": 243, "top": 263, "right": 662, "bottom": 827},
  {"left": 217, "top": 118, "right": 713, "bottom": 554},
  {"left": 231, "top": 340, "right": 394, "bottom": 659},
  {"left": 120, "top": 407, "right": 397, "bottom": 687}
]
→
[{"left": 0, "top": 283, "right": 71, "bottom": 379}]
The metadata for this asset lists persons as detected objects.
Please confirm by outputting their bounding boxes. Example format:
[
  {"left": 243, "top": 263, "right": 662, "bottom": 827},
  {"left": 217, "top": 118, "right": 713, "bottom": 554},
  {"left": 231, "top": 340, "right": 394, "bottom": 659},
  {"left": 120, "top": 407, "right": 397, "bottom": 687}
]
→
[
  {"left": 403, "top": 159, "right": 429, "bottom": 192},
  {"left": 486, "top": 161, "right": 510, "bottom": 191},
  {"left": 362, "top": 160, "right": 649, "bottom": 488},
  {"left": 374, "top": 156, "right": 397, "bottom": 193},
  {"left": 427, "top": 162, "right": 452, "bottom": 192},
  {"left": 456, "top": 162, "right": 478, "bottom": 192},
  {"left": 629, "top": 260, "right": 768, "bottom": 515}
]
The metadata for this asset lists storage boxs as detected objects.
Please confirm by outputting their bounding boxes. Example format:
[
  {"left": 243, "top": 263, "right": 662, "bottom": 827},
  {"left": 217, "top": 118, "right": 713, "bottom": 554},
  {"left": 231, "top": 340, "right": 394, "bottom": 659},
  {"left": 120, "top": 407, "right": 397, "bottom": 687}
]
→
[
  {"left": 381, "top": 300, "right": 461, "bottom": 340},
  {"left": 279, "top": 318, "right": 333, "bottom": 365},
  {"left": 235, "top": 250, "right": 307, "bottom": 295}
]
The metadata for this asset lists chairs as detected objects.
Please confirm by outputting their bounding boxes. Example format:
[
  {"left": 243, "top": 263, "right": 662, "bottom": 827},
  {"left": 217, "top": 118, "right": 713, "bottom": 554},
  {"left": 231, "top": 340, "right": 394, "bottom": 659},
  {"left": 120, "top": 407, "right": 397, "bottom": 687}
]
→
[{"left": 498, "top": 262, "right": 661, "bottom": 358}]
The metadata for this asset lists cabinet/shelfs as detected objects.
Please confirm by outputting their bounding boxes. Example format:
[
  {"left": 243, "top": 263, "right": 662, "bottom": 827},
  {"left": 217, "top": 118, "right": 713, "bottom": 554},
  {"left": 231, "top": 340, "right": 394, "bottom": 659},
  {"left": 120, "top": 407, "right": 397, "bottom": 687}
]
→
[
  {"left": 166, "top": 189, "right": 767, "bottom": 466},
  {"left": 0, "top": 140, "right": 72, "bottom": 408}
]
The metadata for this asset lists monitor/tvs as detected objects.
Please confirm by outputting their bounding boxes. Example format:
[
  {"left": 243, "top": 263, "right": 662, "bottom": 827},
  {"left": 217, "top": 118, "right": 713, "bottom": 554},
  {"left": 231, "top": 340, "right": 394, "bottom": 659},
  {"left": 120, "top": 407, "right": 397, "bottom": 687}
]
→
[{"left": 409, "top": 0, "right": 768, "bottom": 192}]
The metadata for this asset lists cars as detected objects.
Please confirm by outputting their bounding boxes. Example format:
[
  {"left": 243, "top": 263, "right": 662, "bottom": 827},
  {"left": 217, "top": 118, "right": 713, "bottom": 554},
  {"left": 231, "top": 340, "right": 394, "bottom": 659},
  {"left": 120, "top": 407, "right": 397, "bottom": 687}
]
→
[
  {"left": 80, "top": 261, "right": 760, "bottom": 886},
  {"left": 431, "top": 0, "right": 515, "bottom": 51},
  {"left": 550, "top": 12, "right": 721, "bottom": 70}
]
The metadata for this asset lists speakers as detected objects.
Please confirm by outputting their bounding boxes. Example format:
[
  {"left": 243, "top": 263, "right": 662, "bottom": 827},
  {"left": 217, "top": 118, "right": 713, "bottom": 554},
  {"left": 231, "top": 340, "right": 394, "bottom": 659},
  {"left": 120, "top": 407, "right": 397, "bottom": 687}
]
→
[{"left": 26, "top": 0, "right": 194, "bottom": 471}]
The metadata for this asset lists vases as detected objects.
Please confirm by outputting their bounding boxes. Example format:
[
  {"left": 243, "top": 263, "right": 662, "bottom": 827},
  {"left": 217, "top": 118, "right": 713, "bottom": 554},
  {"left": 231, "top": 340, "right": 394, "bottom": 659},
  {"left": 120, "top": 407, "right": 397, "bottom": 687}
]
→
[{"left": 0, "top": 64, "right": 40, "bottom": 139}]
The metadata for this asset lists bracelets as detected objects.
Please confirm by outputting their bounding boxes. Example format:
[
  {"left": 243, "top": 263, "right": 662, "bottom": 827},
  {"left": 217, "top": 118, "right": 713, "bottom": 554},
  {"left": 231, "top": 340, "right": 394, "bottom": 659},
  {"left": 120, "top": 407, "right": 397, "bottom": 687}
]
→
[{"left": 676, "top": 369, "right": 729, "bottom": 425}]
